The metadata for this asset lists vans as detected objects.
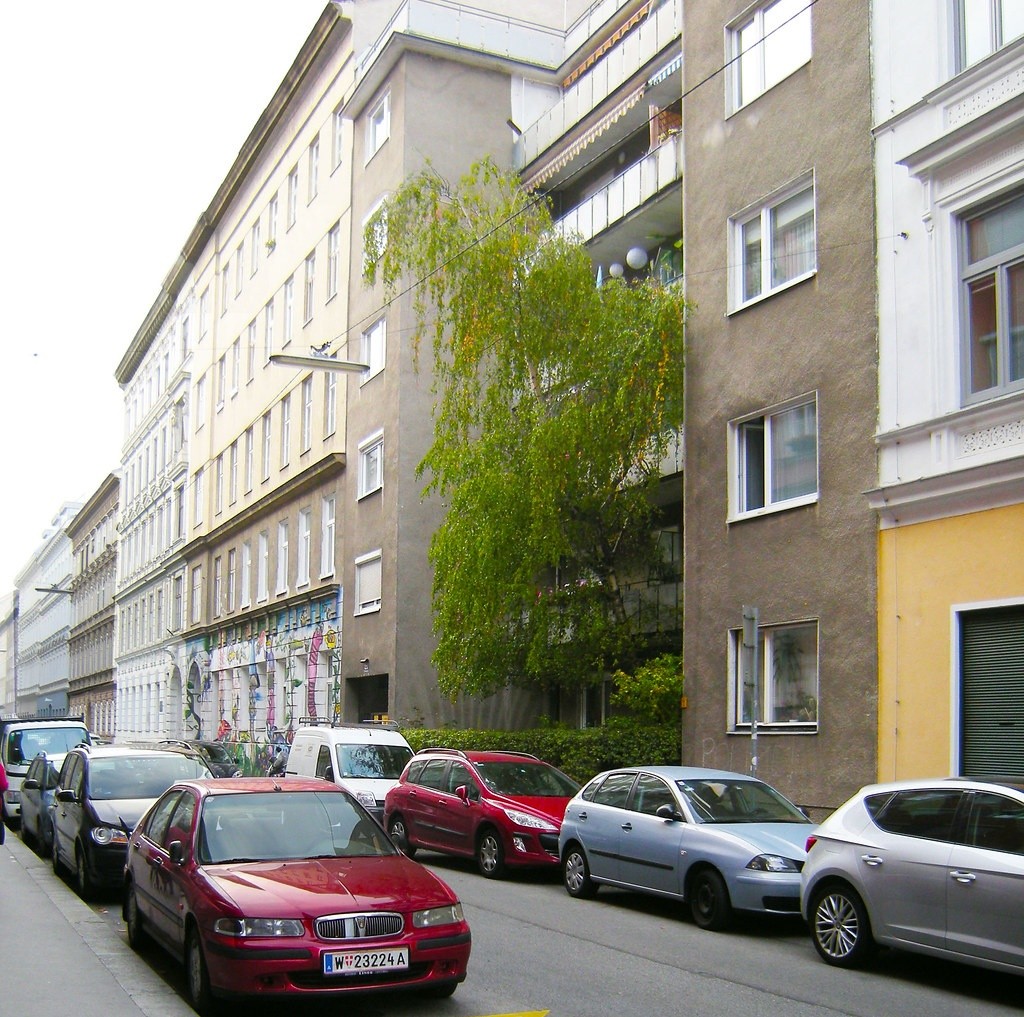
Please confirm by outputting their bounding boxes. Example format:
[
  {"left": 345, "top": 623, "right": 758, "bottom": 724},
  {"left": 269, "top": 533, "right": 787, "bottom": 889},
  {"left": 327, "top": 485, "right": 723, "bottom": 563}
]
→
[{"left": 277, "top": 717, "right": 419, "bottom": 822}]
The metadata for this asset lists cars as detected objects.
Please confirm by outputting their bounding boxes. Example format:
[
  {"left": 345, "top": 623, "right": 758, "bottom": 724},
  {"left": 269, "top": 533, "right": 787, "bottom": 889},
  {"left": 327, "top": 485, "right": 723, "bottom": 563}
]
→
[
  {"left": 799, "top": 772, "right": 1024, "bottom": 989},
  {"left": 121, "top": 776, "right": 472, "bottom": 1015},
  {"left": 88, "top": 732, "right": 101, "bottom": 740},
  {"left": 557, "top": 764, "right": 822, "bottom": 931},
  {"left": 94, "top": 740, "right": 112, "bottom": 745}
]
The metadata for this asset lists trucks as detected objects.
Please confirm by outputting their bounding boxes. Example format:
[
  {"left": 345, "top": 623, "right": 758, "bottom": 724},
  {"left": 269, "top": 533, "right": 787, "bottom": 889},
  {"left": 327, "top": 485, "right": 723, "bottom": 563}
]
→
[{"left": 0, "top": 712, "right": 93, "bottom": 818}]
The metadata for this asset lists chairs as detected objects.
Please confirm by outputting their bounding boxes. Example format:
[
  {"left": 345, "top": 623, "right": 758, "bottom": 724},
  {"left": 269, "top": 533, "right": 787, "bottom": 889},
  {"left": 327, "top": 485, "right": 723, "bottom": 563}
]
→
[{"left": 702, "top": 787, "right": 730, "bottom": 816}]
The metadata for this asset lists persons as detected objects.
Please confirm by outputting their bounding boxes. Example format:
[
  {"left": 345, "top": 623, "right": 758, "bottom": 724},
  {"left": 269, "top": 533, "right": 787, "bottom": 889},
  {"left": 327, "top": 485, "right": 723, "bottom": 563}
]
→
[
  {"left": 273, "top": 747, "right": 289, "bottom": 769},
  {"left": 0, "top": 763, "right": 9, "bottom": 845}
]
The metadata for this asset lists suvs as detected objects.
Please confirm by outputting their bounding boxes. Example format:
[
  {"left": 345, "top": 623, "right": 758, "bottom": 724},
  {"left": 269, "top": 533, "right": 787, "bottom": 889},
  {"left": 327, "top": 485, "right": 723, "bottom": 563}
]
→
[
  {"left": 47, "top": 740, "right": 219, "bottom": 896},
  {"left": 380, "top": 747, "right": 583, "bottom": 879},
  {"left": 157, "top": 739, "right": 243, "bottom": 779},
  {"left": 19, "top": 750, "right": 72, "bottom": 857}
]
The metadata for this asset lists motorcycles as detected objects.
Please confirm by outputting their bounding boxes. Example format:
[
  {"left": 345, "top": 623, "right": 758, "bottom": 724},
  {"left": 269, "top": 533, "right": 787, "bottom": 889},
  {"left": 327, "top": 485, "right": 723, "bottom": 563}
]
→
[{"left": 264, "top": 757, "right": 287, "bottom": 777}]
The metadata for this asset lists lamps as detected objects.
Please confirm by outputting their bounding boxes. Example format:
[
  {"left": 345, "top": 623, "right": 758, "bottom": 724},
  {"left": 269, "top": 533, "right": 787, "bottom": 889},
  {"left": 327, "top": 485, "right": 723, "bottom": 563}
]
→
[{"left": 269, "top": 343, "right": 371, "bottom": 374}]
